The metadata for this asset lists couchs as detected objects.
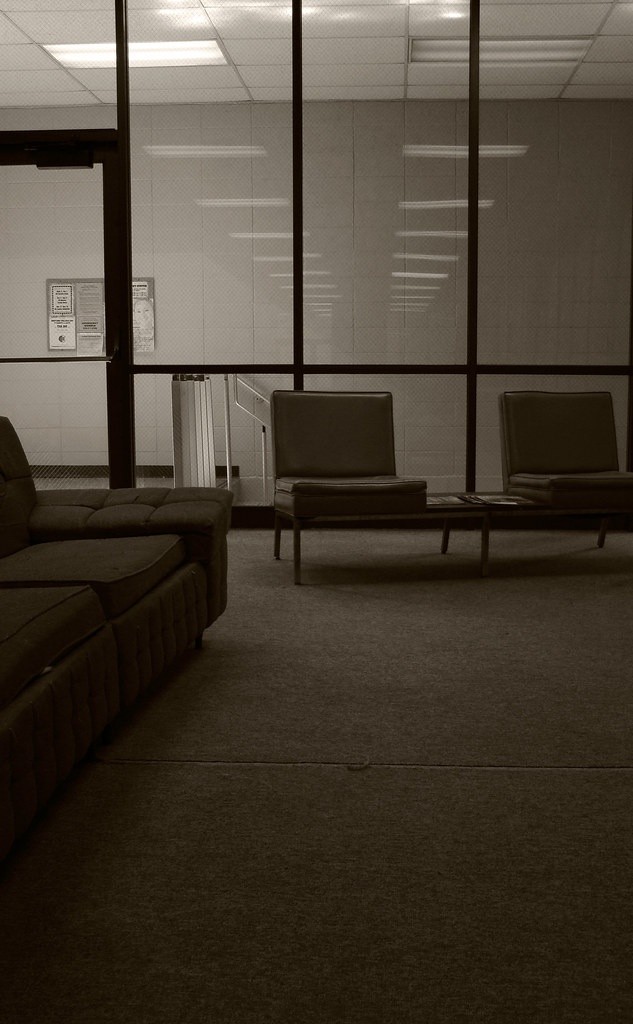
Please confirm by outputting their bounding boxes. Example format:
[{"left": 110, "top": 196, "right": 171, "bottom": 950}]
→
[{"left": 0, "top": 415, "right": 235, "bottom": 874}]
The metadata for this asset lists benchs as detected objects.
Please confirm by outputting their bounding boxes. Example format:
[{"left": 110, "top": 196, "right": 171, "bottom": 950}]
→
[{"left": 271, "top": 389, "right": 633, "bottom": 584}]
